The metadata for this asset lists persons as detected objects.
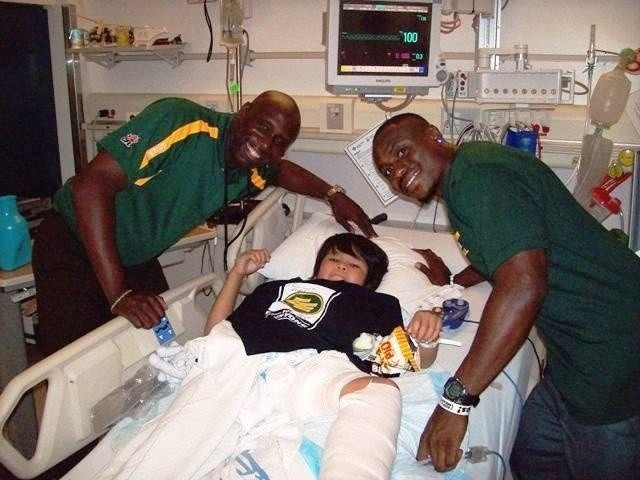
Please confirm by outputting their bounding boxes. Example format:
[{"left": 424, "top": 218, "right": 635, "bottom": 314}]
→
[
  {"left": 190, "top": 232, "right": 443, "bottom": 480},
  {"left": 32, "top": 88, "right": 380, "bottom": 394},
  {"left": 372, "top": 111, "right": 640, "bottom": 480}
]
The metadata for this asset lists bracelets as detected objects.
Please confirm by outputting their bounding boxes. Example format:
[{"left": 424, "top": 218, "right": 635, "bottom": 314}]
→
[
  {"left": 439, "top": 394, "right": 470, "bottom": 417},
  {"left": 110, "top": 287, "right": 132, "bottom": 315},
  {"left": 325, "top": 184, "right": 345, "bottom": 206}
]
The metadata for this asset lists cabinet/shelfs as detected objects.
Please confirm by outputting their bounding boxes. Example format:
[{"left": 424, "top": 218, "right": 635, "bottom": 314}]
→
[{"left": 67, "top": 44, "right": 184, "bottom": 132}]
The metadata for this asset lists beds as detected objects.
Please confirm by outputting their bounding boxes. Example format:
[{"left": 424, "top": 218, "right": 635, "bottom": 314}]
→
[{"left": 0, "top": 185, "right": 535, "bottom": 480}]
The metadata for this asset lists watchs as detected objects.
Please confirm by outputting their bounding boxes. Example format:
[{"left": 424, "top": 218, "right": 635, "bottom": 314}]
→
[{"left": 444, "top": 377, "right": 481, "bottom": 407}]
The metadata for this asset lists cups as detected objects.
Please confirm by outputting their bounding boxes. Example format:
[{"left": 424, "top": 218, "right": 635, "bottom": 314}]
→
[{"left": 69, "top": 29, "right": 90, "bottom": 49}]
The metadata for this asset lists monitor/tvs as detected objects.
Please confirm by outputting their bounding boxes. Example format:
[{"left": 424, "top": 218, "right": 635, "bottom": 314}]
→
[{"left": 326, "top": 1, "right": 449, "bottom": 98}]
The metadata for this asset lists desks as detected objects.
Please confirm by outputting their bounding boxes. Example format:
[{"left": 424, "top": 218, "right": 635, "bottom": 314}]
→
[{"left": 0, "top": 226, "right": 217, "bottom": 439}]
